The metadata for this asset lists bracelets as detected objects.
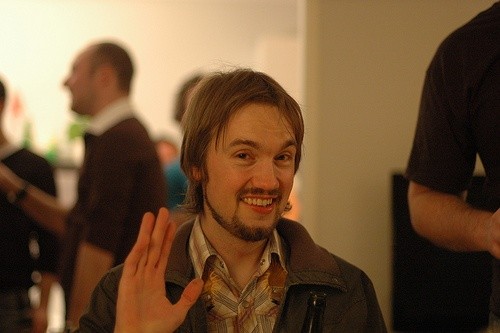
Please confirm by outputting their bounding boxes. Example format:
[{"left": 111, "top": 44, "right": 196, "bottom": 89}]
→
[{"left": 12, "top": 182, "right": 30, "bottom": 207}]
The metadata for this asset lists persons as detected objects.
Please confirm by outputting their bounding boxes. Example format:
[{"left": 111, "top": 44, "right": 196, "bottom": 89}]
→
[
  {"left": 0, "top": 42, "right": 168, "bottom": 333},
  {"left": 0, "top": 79, "right": 59, "bottom": 333},
  {"left": 155, "top": 74, "right": 209, "bottom": 208},
  {"left": 78, "top": 69, "right": 387, "bottom": 333},
  {"left": 405, "top": 2, "right": 500, "bottom": 333}
]
